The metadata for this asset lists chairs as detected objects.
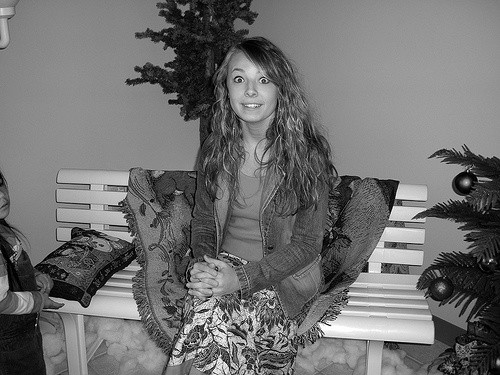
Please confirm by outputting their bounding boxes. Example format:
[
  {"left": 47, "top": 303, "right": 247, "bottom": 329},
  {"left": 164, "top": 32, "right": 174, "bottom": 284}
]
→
[{"left": 47, "top": 168, "right": 436, "bottom": 375}]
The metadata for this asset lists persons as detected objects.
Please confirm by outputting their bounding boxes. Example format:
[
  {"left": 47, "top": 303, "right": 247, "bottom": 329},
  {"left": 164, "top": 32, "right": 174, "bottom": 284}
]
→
[
  {"left": 164, "top": 37, "right": 341, "bottom": 375},
  {"left": 1, "top": 172, "right": 64, "bottom": 375}
]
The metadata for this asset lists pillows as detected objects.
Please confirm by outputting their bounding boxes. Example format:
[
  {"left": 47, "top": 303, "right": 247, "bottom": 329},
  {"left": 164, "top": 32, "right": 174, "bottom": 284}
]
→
[{"left": 35, "top": 226, "right": 153, "bottom": 308}]
